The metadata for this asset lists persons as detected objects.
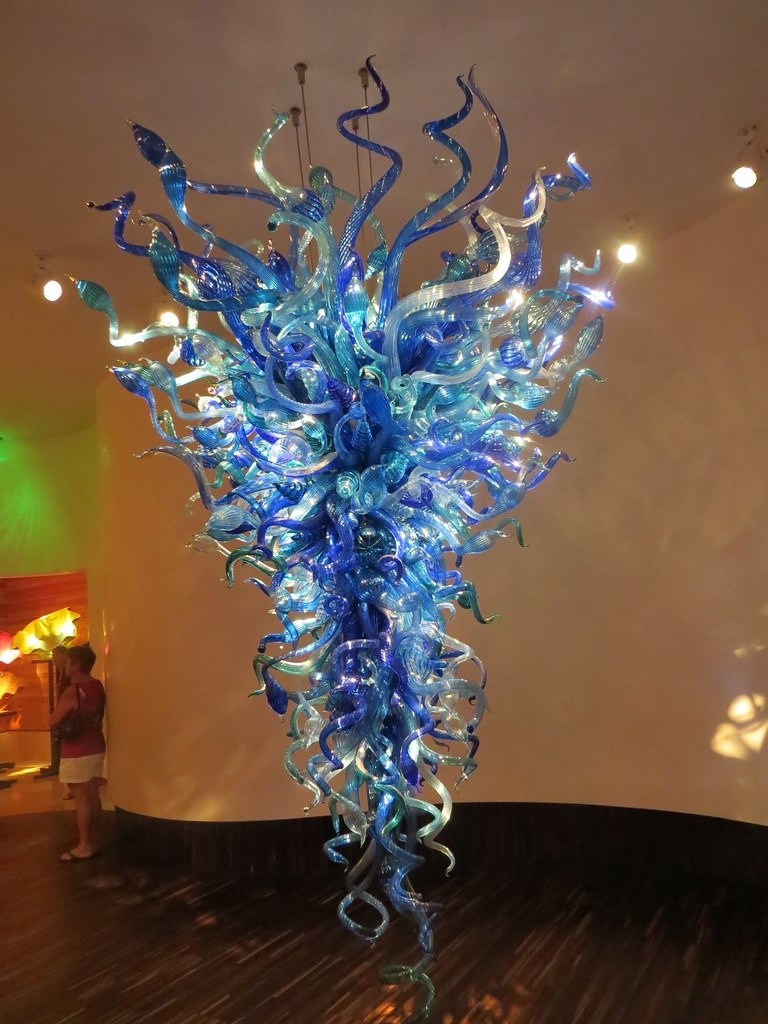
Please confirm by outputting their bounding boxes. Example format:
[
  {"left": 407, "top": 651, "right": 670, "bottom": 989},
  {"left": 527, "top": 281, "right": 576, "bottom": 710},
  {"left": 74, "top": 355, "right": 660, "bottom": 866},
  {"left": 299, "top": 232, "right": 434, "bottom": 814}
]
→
[{"left": 46, "top": 644, "right": 106, "bottom": 862}]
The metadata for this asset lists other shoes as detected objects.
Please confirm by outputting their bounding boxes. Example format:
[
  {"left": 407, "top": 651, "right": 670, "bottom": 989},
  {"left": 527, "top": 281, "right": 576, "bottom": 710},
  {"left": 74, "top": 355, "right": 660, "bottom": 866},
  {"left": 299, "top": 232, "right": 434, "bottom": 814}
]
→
[{"left": 63, "top": 793, "right": 74, "bottom": 800}]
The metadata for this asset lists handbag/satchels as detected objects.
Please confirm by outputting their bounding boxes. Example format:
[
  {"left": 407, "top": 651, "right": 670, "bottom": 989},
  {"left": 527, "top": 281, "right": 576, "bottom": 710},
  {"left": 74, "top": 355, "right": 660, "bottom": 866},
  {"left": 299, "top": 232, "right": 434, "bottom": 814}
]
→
[{"left": 57, "top": 684, "right": 85, "bottom": 741}]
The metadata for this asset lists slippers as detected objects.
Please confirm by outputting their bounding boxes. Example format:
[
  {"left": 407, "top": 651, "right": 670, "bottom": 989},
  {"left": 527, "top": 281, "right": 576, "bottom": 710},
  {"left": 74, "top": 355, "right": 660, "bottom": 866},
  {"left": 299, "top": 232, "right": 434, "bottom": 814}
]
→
[{"left": 59, "top": 851, "right": 101, "bottom": 862}]
